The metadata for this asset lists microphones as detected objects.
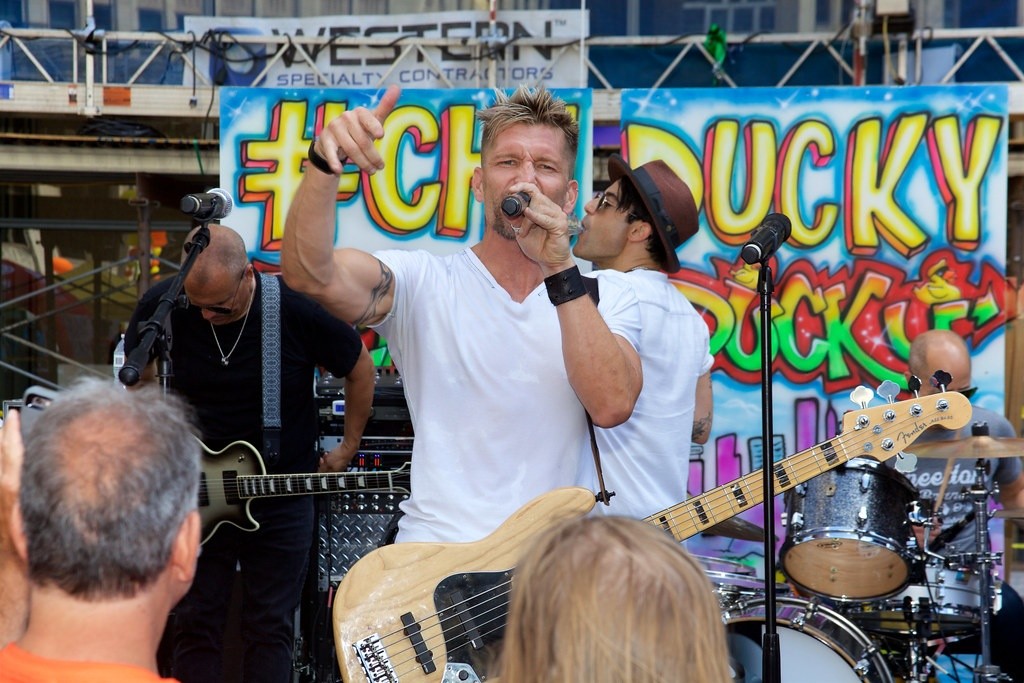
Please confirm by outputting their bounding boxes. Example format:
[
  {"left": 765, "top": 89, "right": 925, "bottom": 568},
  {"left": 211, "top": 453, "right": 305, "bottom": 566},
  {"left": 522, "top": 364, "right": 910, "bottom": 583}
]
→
[
  {"left": 740, "top": 213, "right": 793, "bottom": 264},
  {"left": 929, "top": 509, "right": 977, "bottom": 553},
  {"left": 179, "top": 188, "right": 233, "bottom": 219},
  {"left": 500, "top": 191, "right": 532, "bottom": 218}
]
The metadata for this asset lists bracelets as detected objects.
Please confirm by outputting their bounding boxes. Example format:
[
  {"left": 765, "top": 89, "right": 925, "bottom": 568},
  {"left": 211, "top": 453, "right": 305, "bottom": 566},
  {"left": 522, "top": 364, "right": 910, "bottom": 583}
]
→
[
  {"left": 544, "top": 265, "right": 587, "bottom": 307},
  {"left": 308, "top": 139, "right": 348, "bottom": 175}
]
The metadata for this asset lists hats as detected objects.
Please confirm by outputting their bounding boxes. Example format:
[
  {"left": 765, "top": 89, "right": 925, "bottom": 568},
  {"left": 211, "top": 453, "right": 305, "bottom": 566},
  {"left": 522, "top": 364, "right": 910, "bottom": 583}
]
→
[{"left": 608, "top": 153, "right": 699, "bottom": 273}]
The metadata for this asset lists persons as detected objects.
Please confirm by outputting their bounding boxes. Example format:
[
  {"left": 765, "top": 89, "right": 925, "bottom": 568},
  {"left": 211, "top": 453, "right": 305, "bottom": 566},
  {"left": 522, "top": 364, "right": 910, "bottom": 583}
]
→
[
  {"left": 571, "top": 153, "right": 715, "bottom": 552},
  {"left": 125, "top": 225, "right": 376, "bottom": 683},
  {"left": 885, "top": 331, "right": 1024, "bottom": 683},
  {"left": 280, "top": 84, "right": 643, "bottom": 546},
  {"left": 0, "top": 376, "right": 203, "bottom": 683},
  {"left": 490, "top": 516, "right": 733, "bottom": 683}
]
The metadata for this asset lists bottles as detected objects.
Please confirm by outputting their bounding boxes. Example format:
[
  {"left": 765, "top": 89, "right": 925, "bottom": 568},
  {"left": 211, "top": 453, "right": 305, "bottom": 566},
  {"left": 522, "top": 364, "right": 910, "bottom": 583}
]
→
[{"left": 113, "top": 334, "right": 127, "bottom": 385}]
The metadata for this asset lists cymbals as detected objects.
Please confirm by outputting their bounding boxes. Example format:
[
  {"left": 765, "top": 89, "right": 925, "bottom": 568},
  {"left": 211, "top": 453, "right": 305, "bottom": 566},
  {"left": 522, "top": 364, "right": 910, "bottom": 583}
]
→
[
  {"left": 901, "top": 433, "right": 1024, "bottom": 460},
  {"left": 700, "top": 515, "right": 781, "bottom": 543},
  {"left": 992, "top": 509, "right": 1024, "bottom": 521}
]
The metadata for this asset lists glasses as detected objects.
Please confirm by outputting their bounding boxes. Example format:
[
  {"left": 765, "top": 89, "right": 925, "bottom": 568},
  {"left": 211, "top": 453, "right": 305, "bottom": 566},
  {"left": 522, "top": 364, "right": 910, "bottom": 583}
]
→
[
  {"left": 960, "top": 387, "right": 977, "bottom": 399},
  {"left": 190, "top": 273, "right": 244, "bottom": 315},
  {"left": 594, "top": 191, "right": 637, "bottom": 217}
]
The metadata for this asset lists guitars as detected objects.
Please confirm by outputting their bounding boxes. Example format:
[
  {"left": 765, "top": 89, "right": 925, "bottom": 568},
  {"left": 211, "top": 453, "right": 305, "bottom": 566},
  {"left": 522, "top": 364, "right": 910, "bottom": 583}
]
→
[
  {"left": 183, "top": 429, "right": 414, "bottom": 548},
  {"left": 329, "top": 367, "right": 973, "bottom": 683}
]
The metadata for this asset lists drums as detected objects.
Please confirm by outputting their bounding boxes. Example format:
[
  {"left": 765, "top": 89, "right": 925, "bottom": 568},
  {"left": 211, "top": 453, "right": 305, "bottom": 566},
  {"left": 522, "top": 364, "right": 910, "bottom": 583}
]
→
[
  {"left": 682, "top": 549, "right": 757, "bottom": 580},
  {"left": 777, "top": 455, "right": 920, "bottom": 603},
  {"left": 852, "top": 558, "right": 990, "bottom": 625},
  {"left": 703, "top": 567, "right": 792, "bottom": 604},
  {"left": 718, "top": 592, "right": 896, "bottom": 683}
]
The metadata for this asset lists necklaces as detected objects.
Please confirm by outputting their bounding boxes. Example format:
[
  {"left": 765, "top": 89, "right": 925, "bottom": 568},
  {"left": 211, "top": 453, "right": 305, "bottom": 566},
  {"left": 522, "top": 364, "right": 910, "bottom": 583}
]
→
[
  {"left": 624, "top": 266, "right": 660, "bottom": 273},
  {"left": 211, "top": 273, "right": 256, "bottom": 366}
]
